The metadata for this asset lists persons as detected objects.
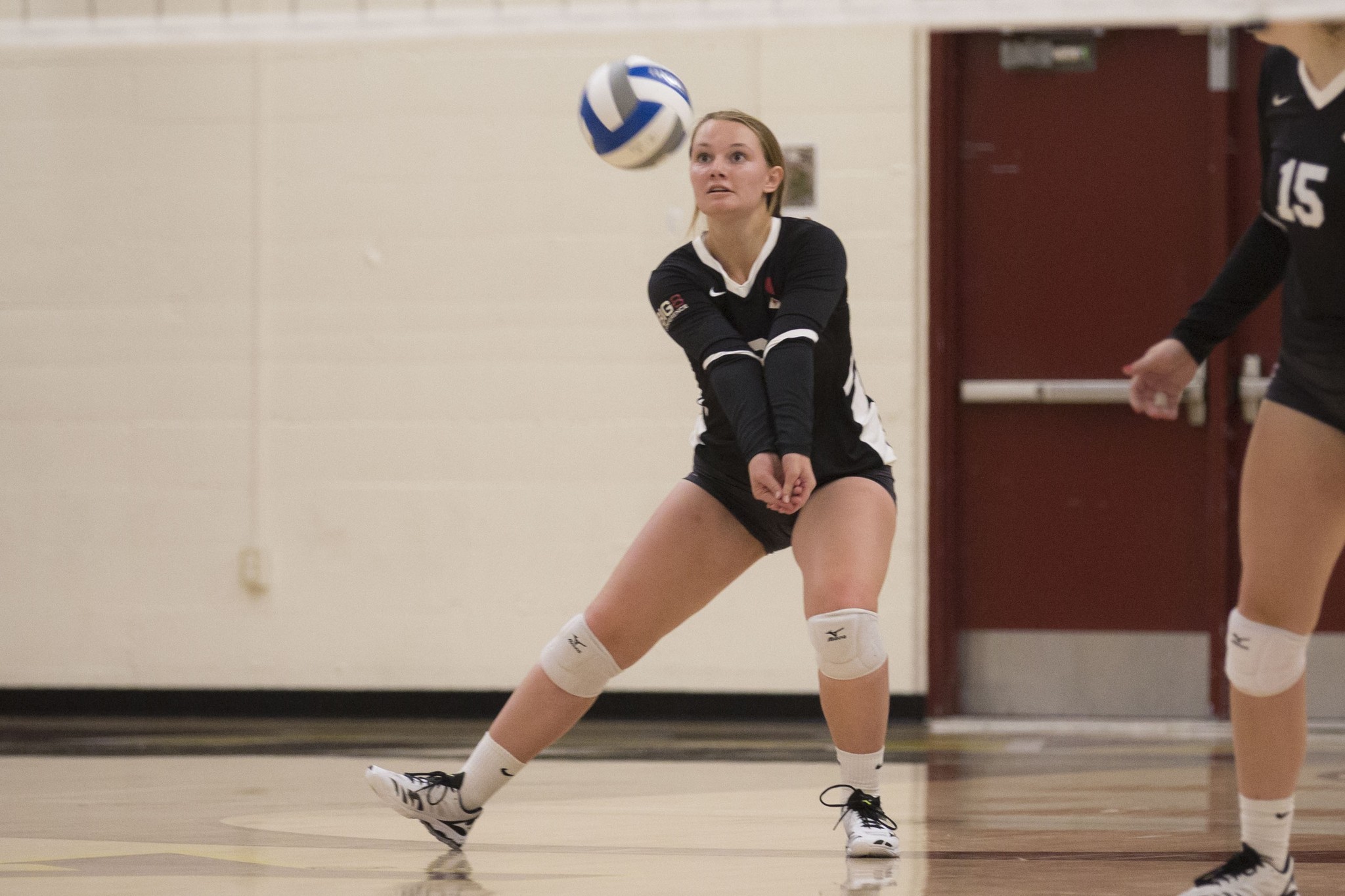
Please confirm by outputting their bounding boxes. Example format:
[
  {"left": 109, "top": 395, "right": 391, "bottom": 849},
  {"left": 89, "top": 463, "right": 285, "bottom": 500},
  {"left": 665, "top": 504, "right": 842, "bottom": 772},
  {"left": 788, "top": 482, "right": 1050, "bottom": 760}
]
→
[
  {"left": 1120, "top": 28, "right": 1345, "bottom": 896},
  {"left": 365, "top": 108, "right": 898, "bottom": 860}
]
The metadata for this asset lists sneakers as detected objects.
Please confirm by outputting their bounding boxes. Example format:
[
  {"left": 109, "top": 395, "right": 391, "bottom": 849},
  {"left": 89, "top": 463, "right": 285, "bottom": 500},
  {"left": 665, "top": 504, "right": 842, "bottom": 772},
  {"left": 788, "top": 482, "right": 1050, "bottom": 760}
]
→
[
  {"left": 366, "top": 764, "right": 484, "bottom": 849},
  {"left": 1176, "top": 841, "right": 1300, "bottom": 896},
  {"left": 820, "top": 784, "right": 900, "bottom": 856}
]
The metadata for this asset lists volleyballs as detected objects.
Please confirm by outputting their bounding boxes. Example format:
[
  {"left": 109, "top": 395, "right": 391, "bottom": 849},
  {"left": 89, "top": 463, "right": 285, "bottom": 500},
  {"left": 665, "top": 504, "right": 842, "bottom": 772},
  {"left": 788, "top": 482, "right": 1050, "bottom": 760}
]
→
[{"left": 577, "top": 55, "right": 693, "bottom": 174}]
